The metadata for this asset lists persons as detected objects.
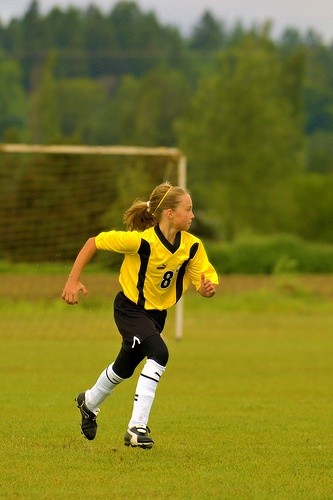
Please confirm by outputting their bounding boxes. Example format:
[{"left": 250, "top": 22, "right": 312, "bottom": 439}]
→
[{"left": 59, "top": 184, "right": 219, "bottom": 451}]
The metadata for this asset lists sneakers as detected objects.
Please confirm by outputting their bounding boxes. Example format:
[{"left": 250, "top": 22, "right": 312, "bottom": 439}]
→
[
  {"left": 124, "top": 426, "right": 154, "bottom": 449},
  {"left": 77, "top": 392, "right": 101, "bottom": 440}
]
